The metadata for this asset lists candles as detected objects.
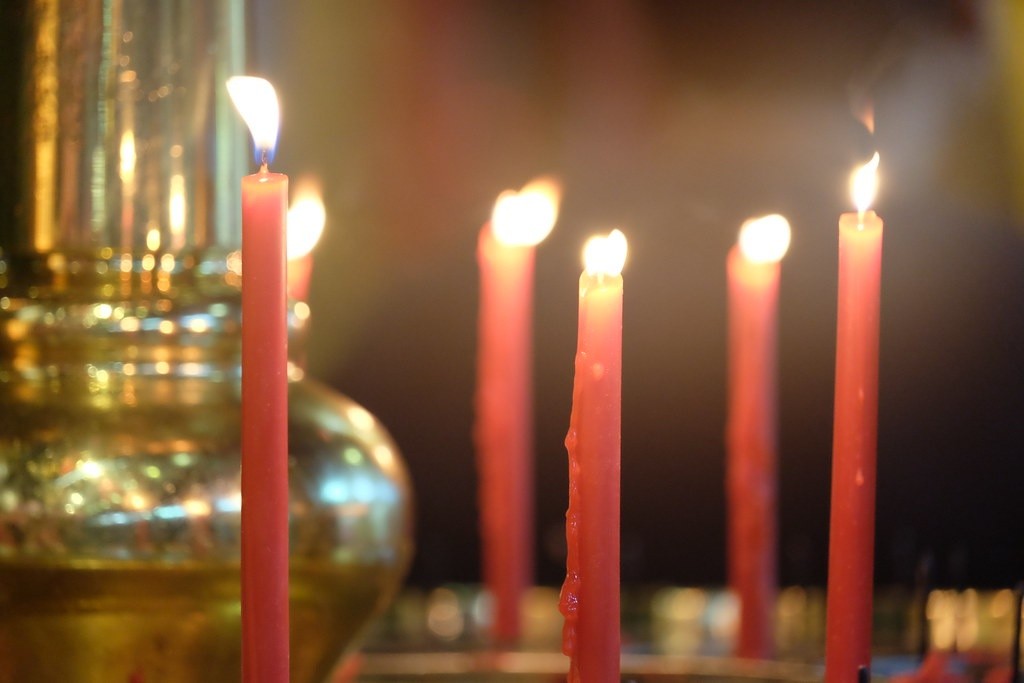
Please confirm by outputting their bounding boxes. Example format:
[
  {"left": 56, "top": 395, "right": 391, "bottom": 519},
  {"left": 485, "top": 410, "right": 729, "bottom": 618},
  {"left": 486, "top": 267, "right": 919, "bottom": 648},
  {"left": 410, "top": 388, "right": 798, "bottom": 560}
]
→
[
  {"left": 224, "top": 64, "right": 288, "bottom": 683},
  {"left": 723, "top": 210, "right": 791, "bottom": 660},
  {"left": 824, "top": 152, "right": 886, "bottom": 683},
  {"left": 474, "top": 176, "right": 564, "bottom": 641},
  {"left": 563, "top": 229, "right": 627, "bottom": 683}
]
